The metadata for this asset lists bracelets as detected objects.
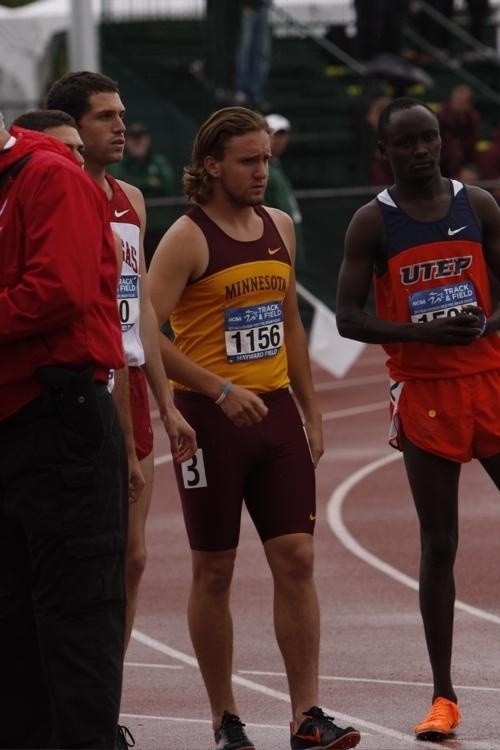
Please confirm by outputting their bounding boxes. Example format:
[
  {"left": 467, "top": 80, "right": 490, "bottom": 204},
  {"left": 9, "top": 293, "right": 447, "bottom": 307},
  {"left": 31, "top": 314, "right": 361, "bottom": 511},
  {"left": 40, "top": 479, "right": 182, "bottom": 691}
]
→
[
  {"left": 215, "top": 382, "right": 231, "bottom": 405},
  {"left": 476, "top": 315, "right": 486, "bottom": 338}
]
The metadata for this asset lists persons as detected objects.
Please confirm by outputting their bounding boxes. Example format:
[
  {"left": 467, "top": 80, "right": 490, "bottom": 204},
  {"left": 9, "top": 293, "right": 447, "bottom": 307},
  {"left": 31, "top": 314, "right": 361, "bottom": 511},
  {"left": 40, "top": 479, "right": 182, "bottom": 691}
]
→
[
  {"left": 263, "top": 115, "right": 305, "bottom": 267},
  {"left": 146, "top": 107, "right": 361, "bottom": 750},
  {"left": 195, "top": 1, "right": 278, "bottom": 116},
  {"left": 105, "top": 118, "right": 185, "bottom": 198},
  {"left": 45, "top": 71, "right": 198, "bottom": 750},
  {"left": 0, "top": 109, "right": 129, "bottom": 750},
  {"left": 335, "top": 99, "right": 500, "bottom": 740},
  {"left": 327, "top": 1, "right": 500, "bottom": 199},
  {"left": 12, "top": 110, "right": 145, "bottom": 504}
]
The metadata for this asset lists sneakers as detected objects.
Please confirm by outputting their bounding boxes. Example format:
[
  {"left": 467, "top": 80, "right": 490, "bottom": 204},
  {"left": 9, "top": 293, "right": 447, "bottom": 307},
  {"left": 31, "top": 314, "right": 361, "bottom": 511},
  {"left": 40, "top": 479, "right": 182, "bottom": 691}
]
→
[
  {"left": 211, "top": 708, "right": 256, "bottom": 749},
  {"left": 412, "top": 697, "right": 463, "bottom": 742},
  {"left": 287, "top": 706, "right": 362, "bottom": 749}
]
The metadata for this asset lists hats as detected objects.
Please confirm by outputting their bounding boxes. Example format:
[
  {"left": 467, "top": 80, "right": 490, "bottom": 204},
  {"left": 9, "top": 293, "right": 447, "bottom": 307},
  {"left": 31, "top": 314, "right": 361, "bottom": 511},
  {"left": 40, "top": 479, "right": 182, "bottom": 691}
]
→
[{"left": 257, "top": 111, "right": 293, "bottom": 134}]
